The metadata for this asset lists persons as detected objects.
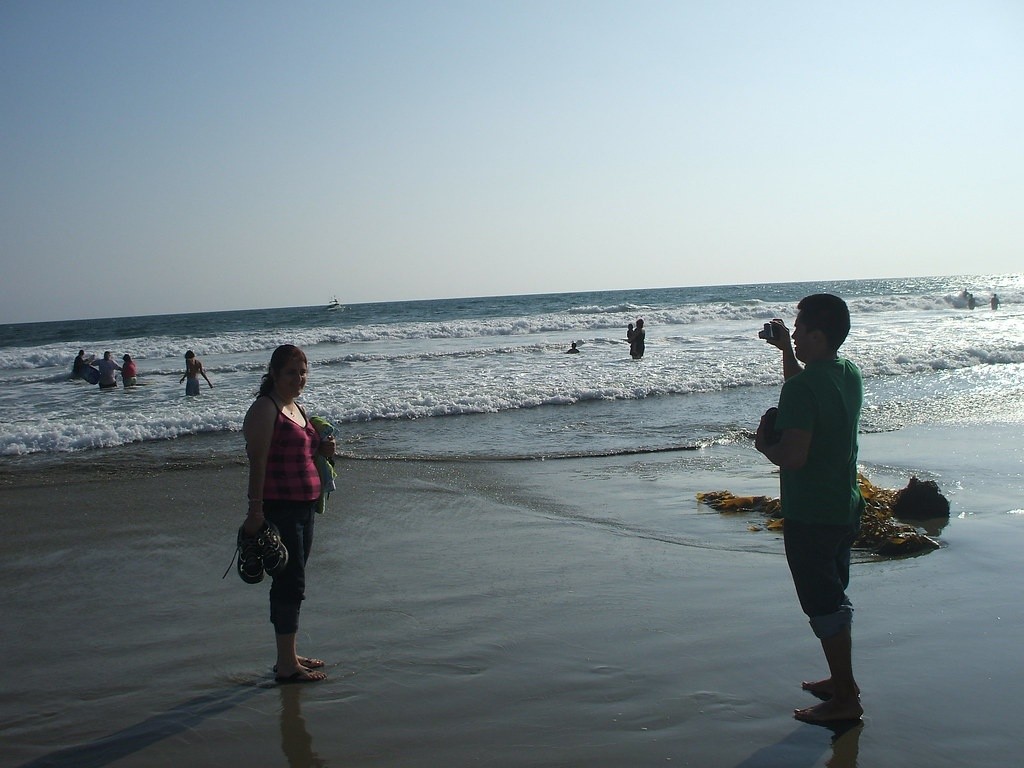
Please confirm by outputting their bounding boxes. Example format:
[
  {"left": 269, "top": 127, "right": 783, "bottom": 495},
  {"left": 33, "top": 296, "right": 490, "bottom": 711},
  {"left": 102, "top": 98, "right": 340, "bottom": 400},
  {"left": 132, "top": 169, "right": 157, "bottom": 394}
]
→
[
  {"left": 244, "top": 345, "right": 335, "bottom": 684},
  {"left": 626, "top": 319, "right": 645, "bottom": 359},
  {"left": 990, "top": 294, "right": 1002, "bottom": 311},
  {"left": 964, "top": 288, "right": 970, "bottom": 297},
  {"left": 567, "top": 343, "right": 579, "bottom": 354},
  {"left": 753, "top": 294, "right": 866, "bottom": 722},
  {"left": 90, "top": 350, "right": 122, "bottom": 388},
  {"left": 73, "top": 350, "right": 90, "bottom": 379},
  {"left": 179, "top": 349, "right": 213, "bottom": 396},
  {"left": 968, "top": 294, "right": 975, "bottom": 310},
  {"left": 122, "top": 355, "right": 137, "bottom": 388}
]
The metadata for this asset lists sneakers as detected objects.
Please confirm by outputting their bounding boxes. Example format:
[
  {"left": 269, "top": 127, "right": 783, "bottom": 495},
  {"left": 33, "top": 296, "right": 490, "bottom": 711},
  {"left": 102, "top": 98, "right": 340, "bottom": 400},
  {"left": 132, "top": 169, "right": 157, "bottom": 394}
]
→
[
  {"left": 257, "top": 521, "right": 289, "bottom": 576},
  {"left": 222, "top": 526, "right": 263, "bottom": 584}
]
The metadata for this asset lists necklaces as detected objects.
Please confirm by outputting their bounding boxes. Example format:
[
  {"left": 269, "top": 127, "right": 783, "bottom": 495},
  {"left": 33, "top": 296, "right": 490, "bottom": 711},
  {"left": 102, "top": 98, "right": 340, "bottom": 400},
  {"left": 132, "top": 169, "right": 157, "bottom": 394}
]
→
[{"left": 290, "top": 407, "right": 295, "bottom": 417}]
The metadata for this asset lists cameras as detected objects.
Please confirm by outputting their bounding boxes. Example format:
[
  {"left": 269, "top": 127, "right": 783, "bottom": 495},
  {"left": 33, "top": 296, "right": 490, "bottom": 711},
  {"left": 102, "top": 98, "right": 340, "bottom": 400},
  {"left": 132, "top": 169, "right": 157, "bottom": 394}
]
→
[{"left": 759, "top": 323, "right": 775, "bottom": 340}]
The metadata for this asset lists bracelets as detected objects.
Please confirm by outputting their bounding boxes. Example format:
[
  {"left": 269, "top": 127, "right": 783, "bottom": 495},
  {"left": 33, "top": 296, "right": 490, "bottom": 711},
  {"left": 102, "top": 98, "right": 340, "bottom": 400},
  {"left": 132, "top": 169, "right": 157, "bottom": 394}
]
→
[{"left": 248, "top": 498, "right": 264, "bottom": 504}]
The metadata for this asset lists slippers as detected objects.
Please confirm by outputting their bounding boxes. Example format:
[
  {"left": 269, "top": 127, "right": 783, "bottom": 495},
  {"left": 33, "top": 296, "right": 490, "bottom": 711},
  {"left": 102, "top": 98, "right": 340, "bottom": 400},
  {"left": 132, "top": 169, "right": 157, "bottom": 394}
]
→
[
  {"left": 275, "top": 667, "right": 326, "bottom": 683},
  {"left": 273, "top": 657, "right": 325, "bottom": 672}
]
